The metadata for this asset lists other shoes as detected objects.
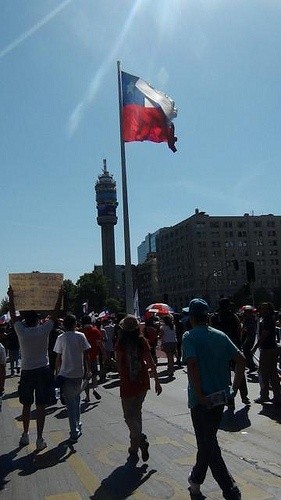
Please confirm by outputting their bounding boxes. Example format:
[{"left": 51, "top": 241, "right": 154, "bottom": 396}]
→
[
  {"left": 93, "top": 391, "right": 101, "bottom": 400},
  {"left": 254, "top": 397, "right": 269, "bottom": 403},
  {"left": 11, "top": 375, "right": 14, "bottom": 378},
  {"left": 81, "top": 398, "right": 90, "bottom": 402},
  {"left": 17, "top": 367, "right": 20, "bottom": 373},
  {"left": 247, "top": 368, "right": 256, "bottom": 374},
  {"left": 141, "top": 440, "right": 149, "bottom": 462},
  {"left": 128, "top": 447, "right": 138, "bottom": 457},
  {"left": 271, "top": 398, "right": 281, "bottom": 403}
]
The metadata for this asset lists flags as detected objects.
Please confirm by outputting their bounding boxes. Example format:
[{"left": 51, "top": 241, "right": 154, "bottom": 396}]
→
[{"left": 121, "top": 73, "right": 179, "bottom": 153}]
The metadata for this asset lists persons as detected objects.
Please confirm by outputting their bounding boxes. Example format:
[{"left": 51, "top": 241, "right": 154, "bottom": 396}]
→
[
  {"left": 52, "top": 314, "right": 93, "bottom": 442},
  {"left": 182, "top": 298, "right": 246, "bottom": 500},
  {"left": 0, "top": 302, "right": 281, "bottom": 411},
  {"left": 115, "top": 316, "right": 162, "bottom": 462},
  {"left": 7, "top": 285, "right": 64, "bottom": 451}
]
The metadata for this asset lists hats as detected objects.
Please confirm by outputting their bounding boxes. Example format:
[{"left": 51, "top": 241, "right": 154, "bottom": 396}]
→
[
  {"left": 214, "top": 298, "right": 234, "bottom": 312},
  {"left": 182, "top": 298, "right": 209, "bottom": 315},
  {"left": 119, "top": 317, "right": 139, "bottom": 331}
]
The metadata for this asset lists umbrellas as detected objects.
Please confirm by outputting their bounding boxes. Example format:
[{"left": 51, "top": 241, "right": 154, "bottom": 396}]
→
[{"left": 145, "top": 303, "right": 174, "bottom": 315}]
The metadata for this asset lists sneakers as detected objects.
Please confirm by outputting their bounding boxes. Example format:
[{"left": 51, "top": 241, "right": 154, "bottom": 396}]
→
[
  {"left": 36, "top": 442, "right": 47, "bottom": 450},
  {"left": 20, "top": 435, "right": 29, "bottom": 445},
  {"left": 188, "top": 475, "right": 201, "bottom": 495}
]
